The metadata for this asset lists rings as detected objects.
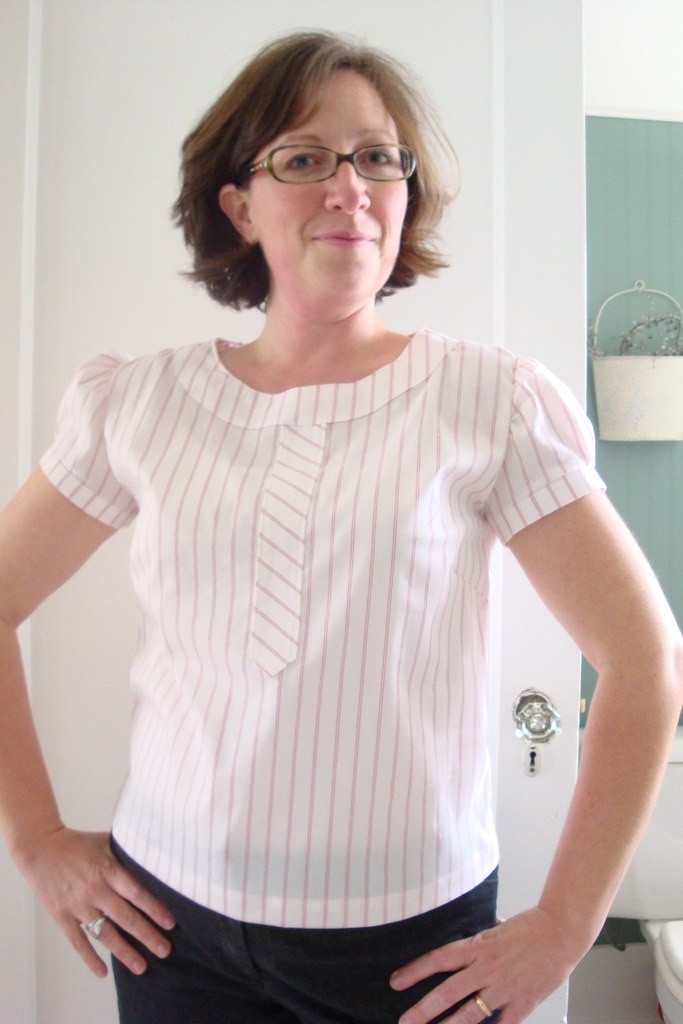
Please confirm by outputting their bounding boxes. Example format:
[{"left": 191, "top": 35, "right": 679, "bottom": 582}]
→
[
  {"left": 475, "top": 996, "right": 493, "bottom": 1017},
  {"left": 86, "top": 914, "right": 106, "bottom": 938}
]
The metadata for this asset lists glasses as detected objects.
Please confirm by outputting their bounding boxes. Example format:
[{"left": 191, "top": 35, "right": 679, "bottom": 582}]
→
[{"left": 246, "top": 142, "right": 415, "bottom": 182}]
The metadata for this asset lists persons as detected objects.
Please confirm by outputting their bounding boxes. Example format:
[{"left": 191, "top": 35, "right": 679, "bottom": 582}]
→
[{"left": 0, "top": 31, "right": 683, "bottom": 1024}]
